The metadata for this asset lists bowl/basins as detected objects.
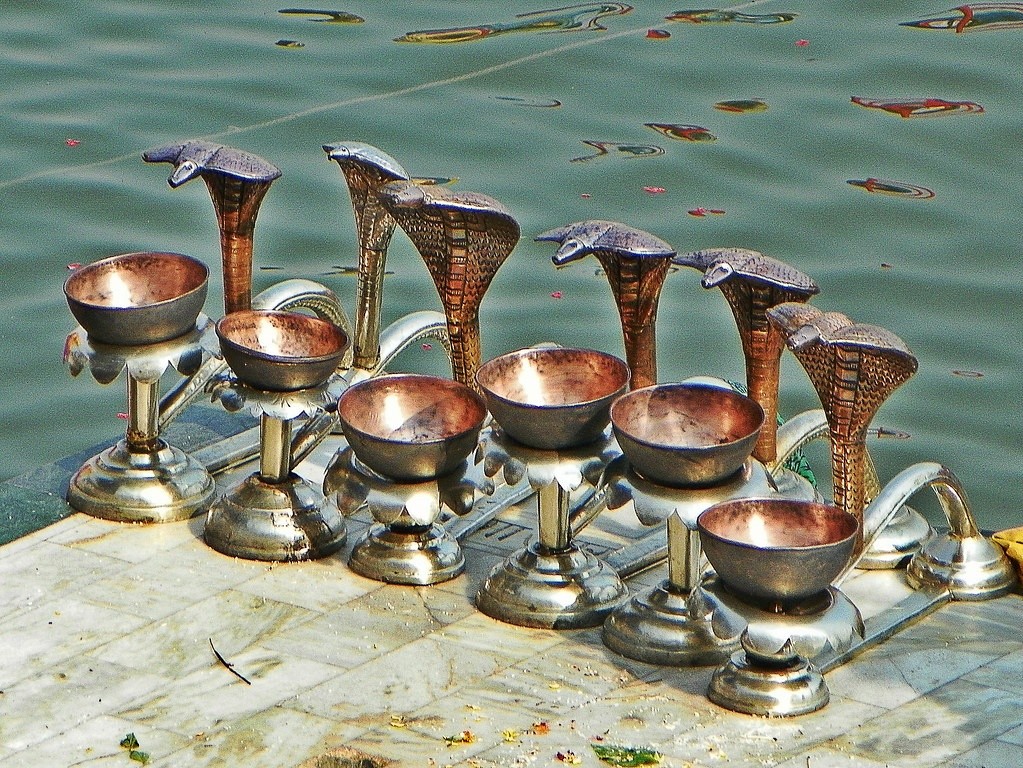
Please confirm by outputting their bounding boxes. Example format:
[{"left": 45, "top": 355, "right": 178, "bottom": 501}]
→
[
  {"left": 216, "top": 311, "right": 351, "bottom": 391},
  {"left": 63, "top": 250, "right": 211, "bottom": 346},
  {"left": 337, "top": 372, "right": 488, "bottom": 480},
  {"left": 609, "top": 382, "right": 766, "bottom": 486},
  {"left": 475, "top": 347, "right": 632, "bottom": 448},
  {"left": 695, "top": 498, "right": 860, "bottom": 603}
]
